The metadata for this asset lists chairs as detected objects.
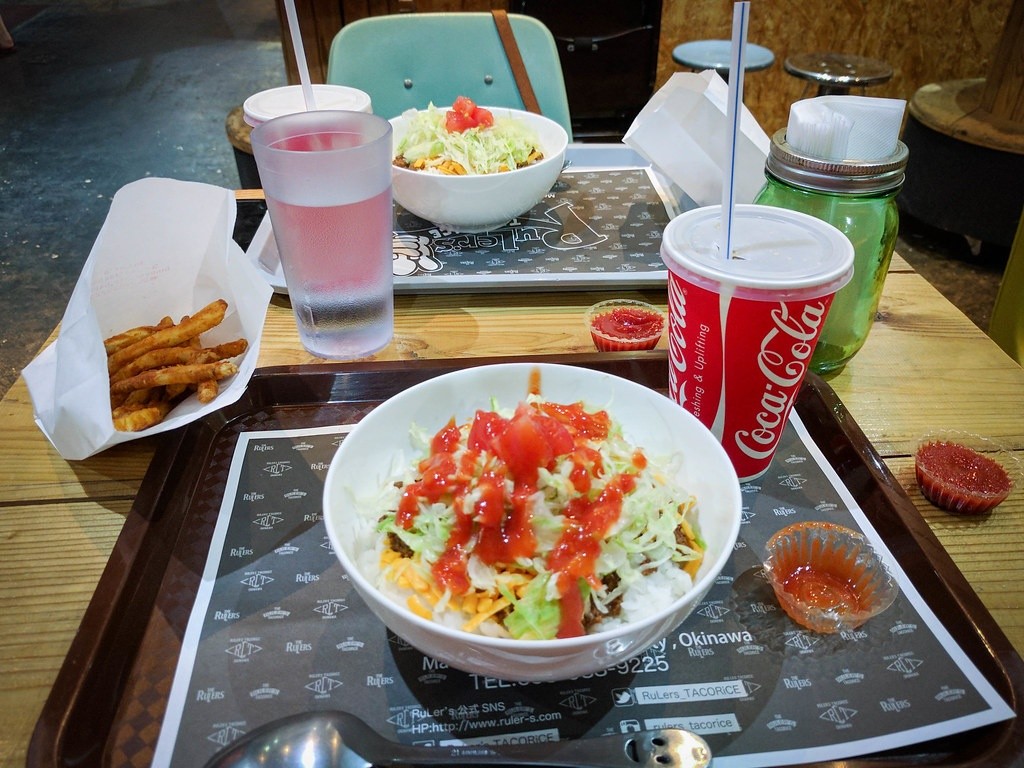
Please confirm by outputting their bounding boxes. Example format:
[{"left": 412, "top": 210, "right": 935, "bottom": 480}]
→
[{"left": 323, "top": 12, "right": 573, "bottom": 147}]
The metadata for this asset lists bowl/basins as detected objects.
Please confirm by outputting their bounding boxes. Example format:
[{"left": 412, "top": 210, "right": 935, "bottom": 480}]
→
[
  {"left": 911, "top": 430, "right": 1023, "bottom": 516},
  {"left": 378, "top": 108, "right": 569, "bottom": 234},
  {"left": 321, "top": 363, "right": 743, "bottom": 683},
  {"left": 585, "top": 299, "right": 667, "bottom": 352},
  {"left": 762, "top": 522, "right": 898, "bottom": 634}
]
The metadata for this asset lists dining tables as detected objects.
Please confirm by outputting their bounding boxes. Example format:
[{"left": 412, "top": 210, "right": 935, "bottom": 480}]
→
[{"left": 2, "top": 250, "right": 1024, "bottom": 768}]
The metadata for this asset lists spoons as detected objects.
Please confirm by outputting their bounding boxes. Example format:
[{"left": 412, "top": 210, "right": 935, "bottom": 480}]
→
[{"left": 205, "top": 710, "right": 711, "bottom": 768}]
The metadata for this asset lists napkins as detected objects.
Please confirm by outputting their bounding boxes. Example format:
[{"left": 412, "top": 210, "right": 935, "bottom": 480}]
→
[{"left": 787, "top": 93, "right": 906, "bottom": 163}]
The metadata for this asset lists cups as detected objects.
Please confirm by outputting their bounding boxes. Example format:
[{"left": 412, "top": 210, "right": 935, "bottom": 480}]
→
[
  {"left": 242, "top": 84, "right": 374, "bottom": 285},
  {"left": 751, "top": 129, "right": 910, "bottom": 377},
  {"left": 661, "top": 206, "right": 856, "bottom": 482},
  {"left": 252, "top": 114, "right": 393, "bottom": 357}
]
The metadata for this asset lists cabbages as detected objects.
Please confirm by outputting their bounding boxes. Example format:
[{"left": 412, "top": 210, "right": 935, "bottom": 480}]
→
[
  {"left": 374, "top": 399, "right": 702, "bottom": 640},
  {"left": 396, "top": 101, "right": 534, "bottom": 175}
]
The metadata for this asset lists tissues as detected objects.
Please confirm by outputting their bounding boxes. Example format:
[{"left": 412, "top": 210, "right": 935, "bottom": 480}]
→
[{"left": 747, "top": 94, "right": 912, "bottom": 371}]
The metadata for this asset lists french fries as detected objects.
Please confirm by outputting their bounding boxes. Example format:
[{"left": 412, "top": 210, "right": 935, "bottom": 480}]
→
[{"left": 103, "top": 298, "right": 250, "bottom": 432}]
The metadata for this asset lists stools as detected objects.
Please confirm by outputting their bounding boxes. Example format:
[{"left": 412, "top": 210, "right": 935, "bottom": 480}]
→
[
  {"left": 783, "top": 52, "right": 893, "bottom": 99},
  {"left": 674, "top": 41, "right": 775, "bottom": 86}
]
